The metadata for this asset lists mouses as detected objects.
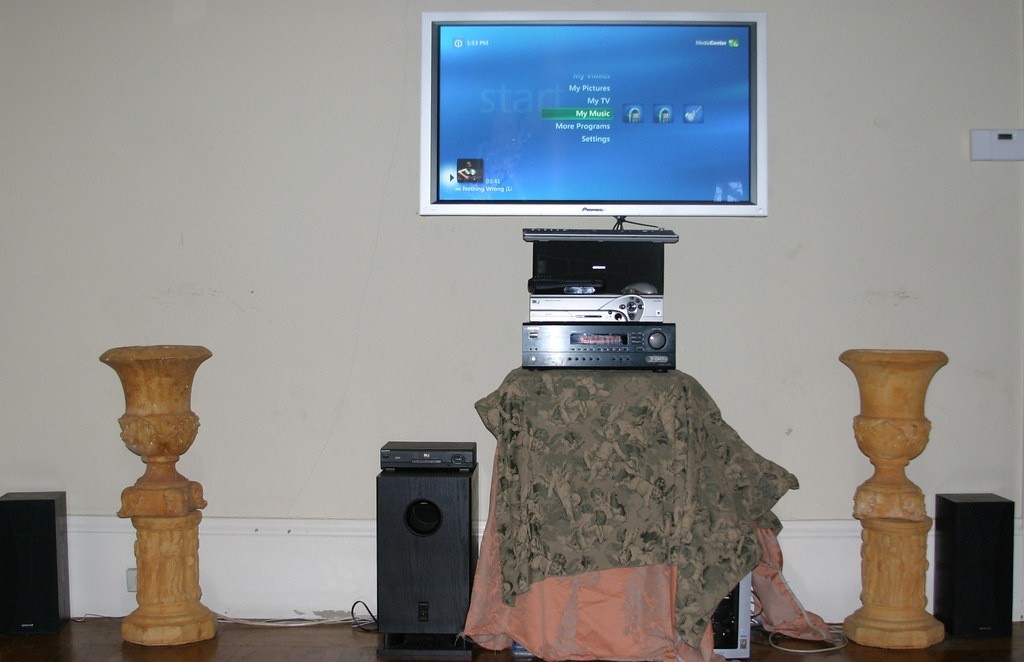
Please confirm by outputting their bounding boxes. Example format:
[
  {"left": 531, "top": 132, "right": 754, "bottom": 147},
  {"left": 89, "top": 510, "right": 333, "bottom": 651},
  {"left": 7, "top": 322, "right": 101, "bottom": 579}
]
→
[{"left": 621, "top": 283, "right": 658, "bottom": 297}]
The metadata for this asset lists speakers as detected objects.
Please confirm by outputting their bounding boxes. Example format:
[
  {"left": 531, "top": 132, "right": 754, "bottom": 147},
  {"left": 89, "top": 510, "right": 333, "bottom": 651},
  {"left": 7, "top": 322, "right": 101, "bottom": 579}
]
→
[
  {"left": 376, "top": 462, "right": 479, "bottom": 636},
  {"left": 0, "top": 491, "right": 70, "bottom": 635},
  {"left": 932, "top": 492, "right": 1015, "bottom": 639},
  {"left": 532, "top": 240, "right": 665, "bottom": 296}
]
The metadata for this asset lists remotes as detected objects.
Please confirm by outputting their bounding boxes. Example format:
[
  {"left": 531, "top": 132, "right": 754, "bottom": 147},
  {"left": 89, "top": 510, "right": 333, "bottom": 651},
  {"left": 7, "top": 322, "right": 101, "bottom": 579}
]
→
[{"left": 528, "top": 277, "right": 606, "bottom": 294}]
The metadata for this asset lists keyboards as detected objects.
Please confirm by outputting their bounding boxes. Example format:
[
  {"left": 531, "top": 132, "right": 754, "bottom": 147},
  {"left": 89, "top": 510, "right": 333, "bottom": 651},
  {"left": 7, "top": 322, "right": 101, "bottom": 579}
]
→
[{"left": 523, "top": 227, "right": 679, "bottom": 244}]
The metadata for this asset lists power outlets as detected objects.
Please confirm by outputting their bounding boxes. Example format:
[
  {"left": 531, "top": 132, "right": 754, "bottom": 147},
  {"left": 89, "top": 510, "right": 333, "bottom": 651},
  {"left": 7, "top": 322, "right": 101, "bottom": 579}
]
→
[{"left": 127, "top": 568, "right": 139, "bottom": 592}]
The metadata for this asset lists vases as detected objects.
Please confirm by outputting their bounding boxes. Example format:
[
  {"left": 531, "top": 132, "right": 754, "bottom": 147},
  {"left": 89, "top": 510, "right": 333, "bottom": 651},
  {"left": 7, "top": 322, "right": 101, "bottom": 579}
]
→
[
  {"left": 98, "top": 345, "right": 213, "bottom": 520},
  {"left": 840, "top": 347, "right": 948, "bottom": 522}
]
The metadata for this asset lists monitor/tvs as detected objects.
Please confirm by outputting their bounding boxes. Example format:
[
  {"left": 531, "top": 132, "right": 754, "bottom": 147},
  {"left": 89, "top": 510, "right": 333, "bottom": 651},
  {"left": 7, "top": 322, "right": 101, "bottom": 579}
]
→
[{"left": 419, "top": 13, "right": 768, "bottom": 217}]
